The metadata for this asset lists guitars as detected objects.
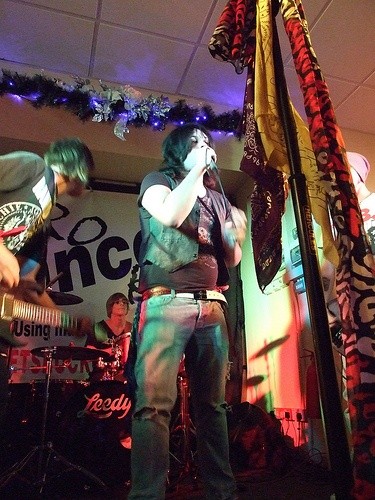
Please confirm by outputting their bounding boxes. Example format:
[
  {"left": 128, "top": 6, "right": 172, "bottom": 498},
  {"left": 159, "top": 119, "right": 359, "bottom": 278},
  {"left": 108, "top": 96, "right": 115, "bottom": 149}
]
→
[{"left": 0, "top": 279, "right": 108, "bottom": 348}]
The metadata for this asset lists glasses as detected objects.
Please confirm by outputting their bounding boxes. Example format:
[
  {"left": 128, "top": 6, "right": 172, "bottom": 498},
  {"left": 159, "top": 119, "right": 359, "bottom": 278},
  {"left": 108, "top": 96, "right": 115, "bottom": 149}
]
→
[{"left": 114, "top": 300, "right": 128, "bottom": 304}]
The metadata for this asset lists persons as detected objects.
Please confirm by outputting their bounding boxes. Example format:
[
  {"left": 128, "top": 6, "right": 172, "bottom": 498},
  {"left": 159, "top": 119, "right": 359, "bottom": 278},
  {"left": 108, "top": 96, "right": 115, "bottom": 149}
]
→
[
  {"left": 83, "top": 292, "right": 135, "bottom": 384},
  {"left": 347, "top": 149, "right": 374, "bottom": 238},
  {"left": 0, "top": 138, "right": 98, "bottom": 337},
  {"left": 129, "top": 124, "right": 246, "bottom": 500}
]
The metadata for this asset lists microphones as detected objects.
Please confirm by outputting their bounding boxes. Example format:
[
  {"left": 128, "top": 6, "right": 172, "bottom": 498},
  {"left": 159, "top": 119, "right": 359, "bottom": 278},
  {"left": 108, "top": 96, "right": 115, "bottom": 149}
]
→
[{"left": 208, "top": 157, "right": 218, "bottom": 172}]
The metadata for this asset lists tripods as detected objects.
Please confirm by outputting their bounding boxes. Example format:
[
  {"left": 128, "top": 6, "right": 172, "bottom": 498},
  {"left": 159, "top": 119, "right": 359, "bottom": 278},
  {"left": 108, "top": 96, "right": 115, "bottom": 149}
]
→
[{"left": 0, "top": 353, "right": 105, "bottom": 500}]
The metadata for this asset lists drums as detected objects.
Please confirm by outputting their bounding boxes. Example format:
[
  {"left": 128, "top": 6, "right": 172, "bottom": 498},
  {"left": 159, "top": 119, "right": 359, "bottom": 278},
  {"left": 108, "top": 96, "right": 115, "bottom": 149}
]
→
[
  {"left": 106, "top": 331, "right": 131, "bottom": 377},
  {"left": 51, "top": 381, "right": 138, "bottom": 488},
  {"left": 179, "top": 353, "right": 231, "bottom": 382},
  {"left": 25, "top": 379, "right": 89, "bottom": 451}
]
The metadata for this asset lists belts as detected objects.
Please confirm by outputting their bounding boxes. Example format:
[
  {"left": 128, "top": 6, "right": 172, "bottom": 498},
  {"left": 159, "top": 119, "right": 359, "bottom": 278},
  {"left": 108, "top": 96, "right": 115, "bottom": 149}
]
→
[{"left": 159, "top": 289, "right": 229, "bottom": 304}]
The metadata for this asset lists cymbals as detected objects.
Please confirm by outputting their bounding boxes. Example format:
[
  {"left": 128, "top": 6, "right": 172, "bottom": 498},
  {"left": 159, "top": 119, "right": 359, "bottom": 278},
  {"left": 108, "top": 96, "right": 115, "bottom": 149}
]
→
[
  {"left": 30, "top": 346, "right": 110, "bottom": 361},
  {"left": 45, "top": 289, "right": 84, "bottom": 306}
]
